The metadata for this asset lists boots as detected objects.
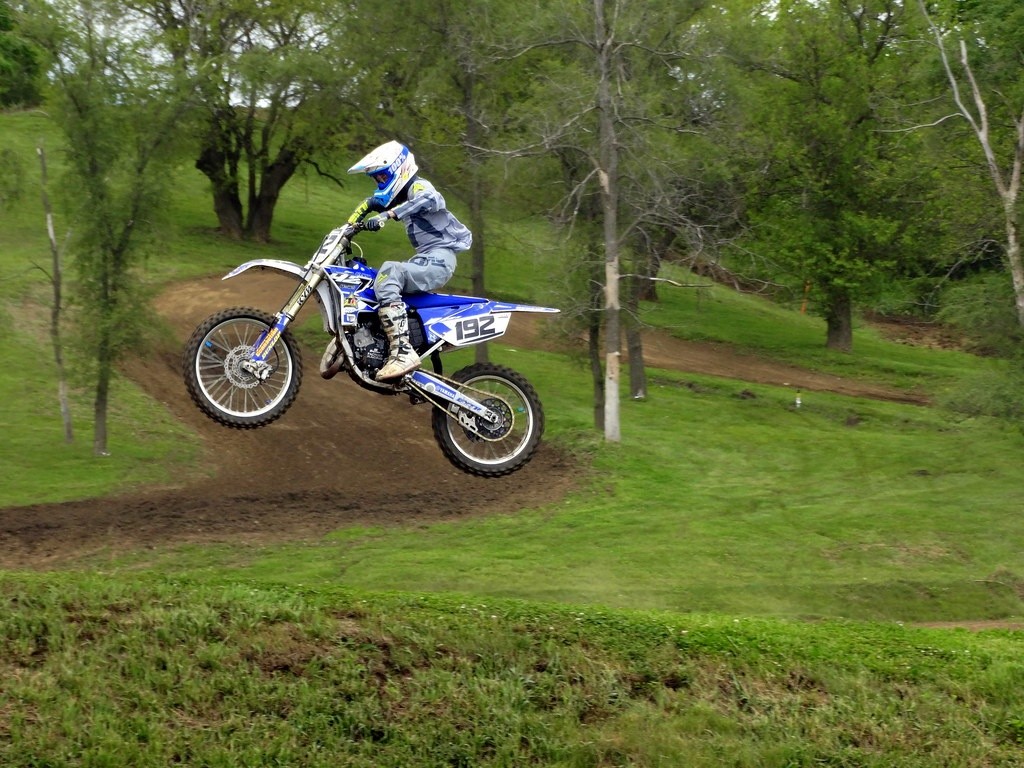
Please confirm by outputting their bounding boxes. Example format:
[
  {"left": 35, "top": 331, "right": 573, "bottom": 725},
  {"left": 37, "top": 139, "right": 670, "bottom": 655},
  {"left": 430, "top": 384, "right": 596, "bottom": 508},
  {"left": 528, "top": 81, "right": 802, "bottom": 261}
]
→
[{"left": 375, "top": 301, "right": 421, "bottom": 380}]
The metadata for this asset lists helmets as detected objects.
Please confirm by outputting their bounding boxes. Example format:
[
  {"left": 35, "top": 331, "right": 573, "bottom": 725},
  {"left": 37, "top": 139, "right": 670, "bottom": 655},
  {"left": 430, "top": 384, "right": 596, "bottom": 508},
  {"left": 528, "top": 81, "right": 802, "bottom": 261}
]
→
[{"left": 347, "top": 140, "right": 419, "bottom": 208}]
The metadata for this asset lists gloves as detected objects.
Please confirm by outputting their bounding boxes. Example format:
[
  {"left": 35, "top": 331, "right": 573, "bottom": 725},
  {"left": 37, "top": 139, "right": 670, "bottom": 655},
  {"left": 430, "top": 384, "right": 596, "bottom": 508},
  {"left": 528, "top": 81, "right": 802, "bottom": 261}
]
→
[{"left": 365, "top": 211, "right": 391, "bottom": 231}]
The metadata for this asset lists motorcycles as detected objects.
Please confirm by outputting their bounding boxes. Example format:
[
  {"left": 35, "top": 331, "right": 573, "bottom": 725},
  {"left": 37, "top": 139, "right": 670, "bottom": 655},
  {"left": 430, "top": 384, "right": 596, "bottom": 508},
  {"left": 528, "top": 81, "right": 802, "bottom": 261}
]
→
[{"left": 180, "top": 223, "right": 563, "bottom": 480}]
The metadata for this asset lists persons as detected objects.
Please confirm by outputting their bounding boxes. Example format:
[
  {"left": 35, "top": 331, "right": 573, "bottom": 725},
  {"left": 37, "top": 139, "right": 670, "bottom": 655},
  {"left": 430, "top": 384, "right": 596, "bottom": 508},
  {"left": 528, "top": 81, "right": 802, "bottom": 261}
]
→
[{"left": 338, "top": 140, "right": 472, "bottom": 379}]
